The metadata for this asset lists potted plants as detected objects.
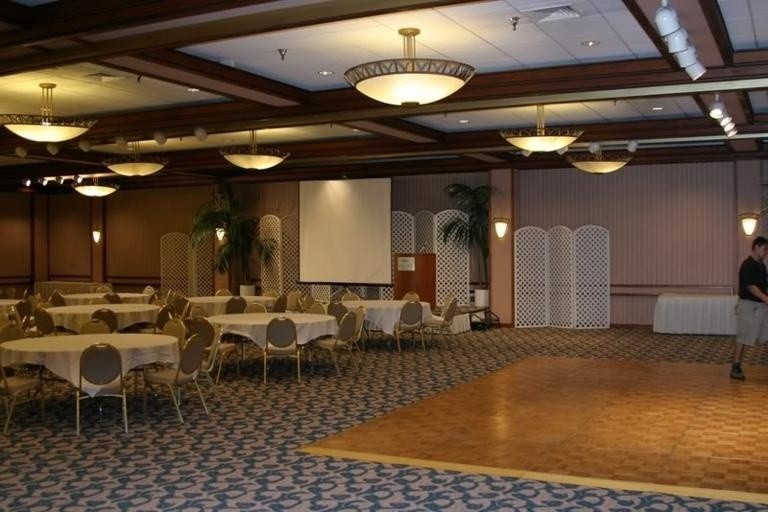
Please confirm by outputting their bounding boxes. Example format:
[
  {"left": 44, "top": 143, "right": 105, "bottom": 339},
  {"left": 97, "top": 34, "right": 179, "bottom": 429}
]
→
[
  {"left": 191, "top": 188, "right": 274, "bottom": 299},
  {"left": 440, "top": 183, "right": 491, "bottom": 319}
]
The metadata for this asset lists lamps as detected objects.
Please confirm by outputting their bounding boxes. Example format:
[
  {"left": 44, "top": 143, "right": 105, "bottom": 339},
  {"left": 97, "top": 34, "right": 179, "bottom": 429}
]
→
[
  {"left": 46, "top": 143, "right": 61, "bottom": 157},
  {"left": 4, "top": 83, "right": 97, "bottom": 144},
  {"left": 718, "top": 112, "right": 738, "bottom": 138},
  {"left": 343, "top": 28, "right": 477, "bottom": 107},
  {"left": 37, "top": 177, "right": 47, "bottom": 187},
  {"left": 672, "top": 45, "right": 698, "bottom": 69},
  {"left": 21, "top": 177, "right": 32, "bottom": 188},
  {"left": 106, "top": 136, "right": 165, "bottom": 179},
  {"left": 565, "top": 145, "right": 633, "bottom": 175},
  {"left": 74, "top": 175, "right": 116, "bottom": 204},
  {"left": 684, "top": 62, "right": 707, "bottom": 82},
  {"left": 72, "top": 174, "right": 79, "bottom": 184},
  {"left": 54, "top": 176, "right": 64, "bottom": 185},
  {"left": 221, "top": 130, "right": 291, "bottom": 172},
  {"left": 14, "top": 146, "right": 28, "bottom": 157},
  {"left": 738, "top": 212, "right": 760, "bottom": 238},
  {"left": 664, "top": 29, "right": 689, "bottom": 54},
  {"left": 493, "top": 217, "right": 511, "bottom": 240},
  {"left": 504, "top": 103, "right": 585, "bottom": 154},
  {"left": 708, "top": 91, "right": 723, "bottom": 119},
  {"left": 90, "top": 226, "right": 103, "bottom": 245},
  {"left": 652, "top": 0, "right": 679, "bottom": 37}
]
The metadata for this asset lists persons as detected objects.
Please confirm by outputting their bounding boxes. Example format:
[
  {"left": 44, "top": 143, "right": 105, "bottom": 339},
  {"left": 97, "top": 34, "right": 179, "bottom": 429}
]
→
[{"left": 730, "top": 236, "right": 768, "bottom": 379}]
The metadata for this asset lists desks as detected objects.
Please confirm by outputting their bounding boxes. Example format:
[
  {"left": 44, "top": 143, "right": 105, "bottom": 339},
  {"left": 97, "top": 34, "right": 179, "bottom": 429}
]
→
[{"left": 656, "top": 294, "right": 738, "bottom": 337}]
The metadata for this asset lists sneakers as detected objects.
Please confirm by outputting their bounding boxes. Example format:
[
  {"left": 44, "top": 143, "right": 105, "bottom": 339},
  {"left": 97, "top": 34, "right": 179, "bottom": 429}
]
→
[{"left": 729, "top": 367, "right": 745, "bottom": 380}]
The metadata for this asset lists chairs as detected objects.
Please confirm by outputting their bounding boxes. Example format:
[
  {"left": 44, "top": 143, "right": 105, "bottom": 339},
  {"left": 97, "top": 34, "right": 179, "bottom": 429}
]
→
[
  {"left": 224, "top": 297, "right": 245, "bottom": 315},
  {"left": 146, "top": 291, "right": 159, "bottom": 305},
  {"left": 263, "top": 317, "right": 302, "bottom": 383},
  {"left": 52, "top": 291, "right": 66, "bottom": 308},
  {"left": 80, "top": 319, "right": 111, "bottom": 336},
  {"left": 142, "top": 319, "right": 183, "bottom": 395},
  {"left": 295, "top": 297, "right": 308, "bottom": 315},
  {"left": 273, "top": 295, "right": 291, "bottom": 316},
  {"left": 341, "top": 292, "right": 361, "bottom": 303},
  {"left": 0, "top": 304, "right": 42, "bottom": 393},
  {"left": 327, "top": 302, "right": 348, "bottom": 338},
  {"left": 103, "top": 293, "right": 121, "bottom": 305},
  {"left": 304, "top": 295, "right": 315, "bottom": 310},
  {"left": 214, "top": 288, "right": 233, "bottom": 298},
  {"left": 142, "top": 333, "right": 209, "bottom": 425},
  {"left": 142, "top": 285, "right": 157, "bottom": 302},
  {"left": 322, "top": 304, "right": 366, "bottom": 366},
  {"left": 91, "top": 307, "right": 116, "bottom": 333},
  {"left": 402, "top": 290, "right": 420, "bottom": 303},
  {"left": 35, "top": 309, "right": 59, "bottom": 337},
  {"left": 309, "top": 312, "right": 357, "bottom": 376},
  {"left": 162, "top": 319, "right": 187, "bottom": 364},
  {"left": 424, "top": 298, "right": 459, "bottom": 350},
  {"left": 0, "top": 357, "right": 47, "bottom": 434},
  {"left": 190, "top": 305, "right": 240, "bottom": 384},
  {"left": 118, "top": 320, "right": 158, "bottom": 336},
  {"left": 393, "top": 301, "right": 427, "bottom": 353},
  {"left": 75, "top": 343, "right": 128, "bottom": 435},
  {"left": 244, "top": 304, "right": 268, "bottom": 315},
  {"left": 188, "top": 316, "right": 241, "bottom": 382}
]
